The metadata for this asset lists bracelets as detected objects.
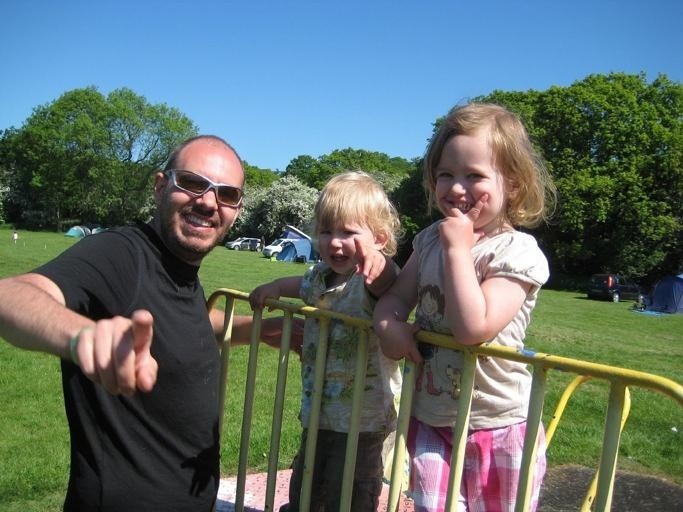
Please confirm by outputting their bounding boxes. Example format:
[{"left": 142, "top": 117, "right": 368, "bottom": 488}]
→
[{"left": 69, "top": 322, "right": 96, "bottom": 368}]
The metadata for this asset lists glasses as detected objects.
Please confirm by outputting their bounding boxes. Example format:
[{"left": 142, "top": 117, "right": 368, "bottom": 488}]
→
[{"left": 165, "top": 167, "right": 245, "bottom": 208}]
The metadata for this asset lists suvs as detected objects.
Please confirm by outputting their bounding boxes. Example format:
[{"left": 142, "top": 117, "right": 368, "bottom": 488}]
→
[{"left": 587, "top": 268, "right": 647, "bottom": 307}]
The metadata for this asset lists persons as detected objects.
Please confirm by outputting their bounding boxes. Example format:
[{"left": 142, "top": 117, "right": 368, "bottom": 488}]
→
[
  {"left": 249, "top": 165, "right": 403, "bottom": 512},
  {"left": 0, "top": 136, "right": 305, "bottom": 512},
  {"left": 374, "top": 97, "right": 550, "bottom": 512}
]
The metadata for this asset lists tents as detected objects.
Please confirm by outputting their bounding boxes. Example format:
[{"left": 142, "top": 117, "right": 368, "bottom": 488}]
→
[
  {"left": 645, "top": 274, "right": 683, "bottom": 312},
  {"left": 275, "top": 239, "right": 314, "bottom": 262},
  {"left": 64, "top": 224, "right": 86, "bottom": 237}
]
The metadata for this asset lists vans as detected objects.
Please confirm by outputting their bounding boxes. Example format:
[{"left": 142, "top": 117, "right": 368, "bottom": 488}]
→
[
  {"left": 262, "top": 239, "right": 300, "bottom": 258},
  {"left": 226, "top": 235, "right": 262, "bottom": 251}
]
